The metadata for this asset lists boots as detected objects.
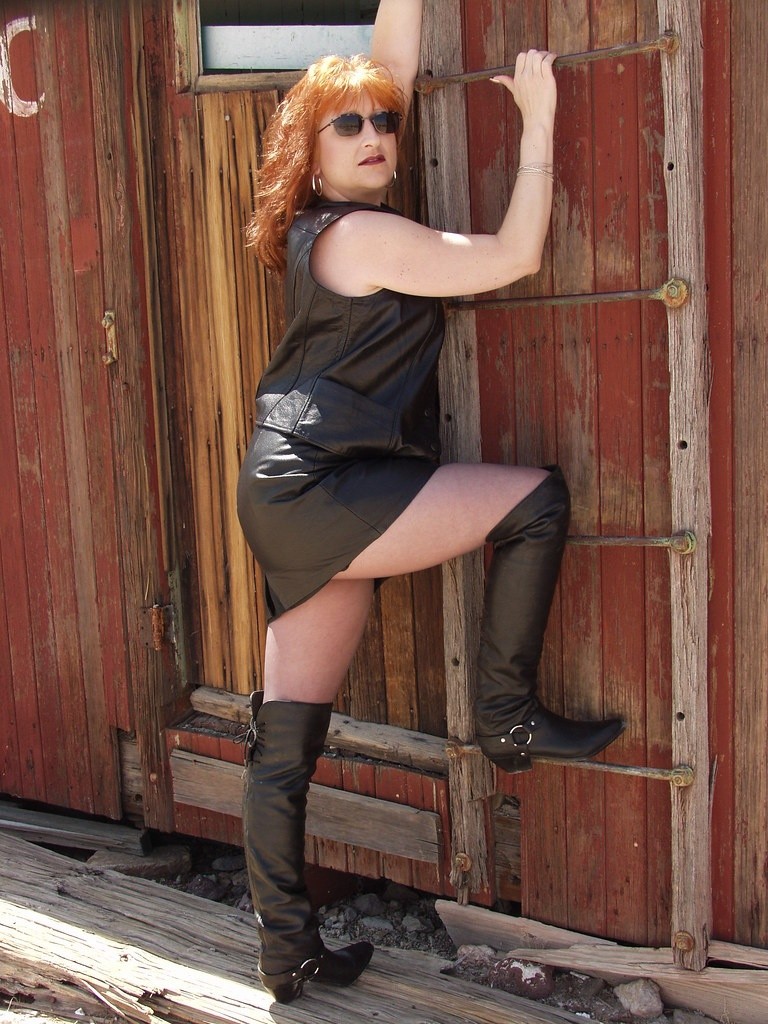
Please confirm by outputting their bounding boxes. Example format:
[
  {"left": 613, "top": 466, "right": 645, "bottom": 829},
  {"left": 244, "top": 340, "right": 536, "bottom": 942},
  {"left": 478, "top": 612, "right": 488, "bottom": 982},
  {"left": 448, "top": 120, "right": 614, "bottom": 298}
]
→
[
  {"left": 244, "top": 690, "right": 373, "bottom": 1004},
  {"left": 476, "top": 465, "right": 624, "bottom": 773}
]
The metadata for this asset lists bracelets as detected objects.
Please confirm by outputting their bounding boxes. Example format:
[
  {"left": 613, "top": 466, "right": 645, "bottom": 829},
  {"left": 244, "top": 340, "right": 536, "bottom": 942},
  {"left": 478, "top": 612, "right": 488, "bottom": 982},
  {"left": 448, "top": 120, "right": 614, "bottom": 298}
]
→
[{"left": 517, "top": 162, "right": 555, "bottom": 181}]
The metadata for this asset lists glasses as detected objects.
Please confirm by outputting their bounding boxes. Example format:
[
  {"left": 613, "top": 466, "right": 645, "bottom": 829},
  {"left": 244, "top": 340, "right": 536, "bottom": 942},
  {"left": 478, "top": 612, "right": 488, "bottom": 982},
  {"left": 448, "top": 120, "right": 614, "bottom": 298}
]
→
[{"left": 316, "top": 110, "right": 403, "bottom": 136}]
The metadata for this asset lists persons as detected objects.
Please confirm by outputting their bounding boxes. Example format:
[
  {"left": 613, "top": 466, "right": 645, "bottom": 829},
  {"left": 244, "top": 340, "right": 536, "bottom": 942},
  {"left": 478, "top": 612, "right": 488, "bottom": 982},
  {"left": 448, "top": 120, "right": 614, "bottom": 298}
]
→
[{"left": 237, "top": 0, "right": 627, "bottom": 1005}]
